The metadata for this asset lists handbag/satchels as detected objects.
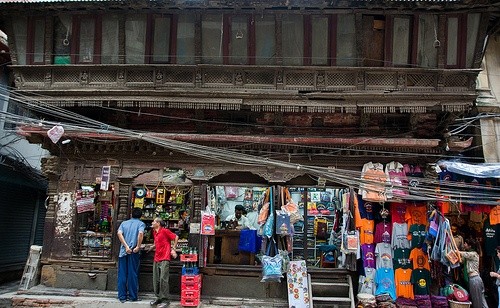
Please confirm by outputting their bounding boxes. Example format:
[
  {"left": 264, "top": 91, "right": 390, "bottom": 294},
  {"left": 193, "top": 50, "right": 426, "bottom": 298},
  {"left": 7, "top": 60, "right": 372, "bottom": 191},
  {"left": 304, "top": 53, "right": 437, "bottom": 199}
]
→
[
  {"left": 451, "top": 284, "right": 469, "bottom": 302},
  {"left": 445, "top": 249, "right": 458, "bottom": 265},
  {"left": 238, "top": 227, "right": 259, "bottom": 252}
]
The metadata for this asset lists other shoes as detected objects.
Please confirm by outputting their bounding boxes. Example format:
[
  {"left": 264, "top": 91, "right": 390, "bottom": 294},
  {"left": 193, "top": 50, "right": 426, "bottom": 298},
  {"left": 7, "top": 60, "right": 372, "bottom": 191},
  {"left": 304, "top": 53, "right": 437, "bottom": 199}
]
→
[
  {"left": 150, "top": 298, "right": 163, "bottom": 306},
  {"left": 120, "top": 299, "right": 126, "bottom": 303},
  {"left": 157, "top": 300, "right": 169, "bottom": 308}
]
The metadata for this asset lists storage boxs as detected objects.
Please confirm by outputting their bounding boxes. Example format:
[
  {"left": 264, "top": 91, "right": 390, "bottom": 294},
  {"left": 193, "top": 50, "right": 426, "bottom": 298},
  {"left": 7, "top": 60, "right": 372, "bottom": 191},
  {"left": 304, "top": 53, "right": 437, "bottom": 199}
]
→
[{"left": 178, "top": 253, "right": 203, "bottom": 307}]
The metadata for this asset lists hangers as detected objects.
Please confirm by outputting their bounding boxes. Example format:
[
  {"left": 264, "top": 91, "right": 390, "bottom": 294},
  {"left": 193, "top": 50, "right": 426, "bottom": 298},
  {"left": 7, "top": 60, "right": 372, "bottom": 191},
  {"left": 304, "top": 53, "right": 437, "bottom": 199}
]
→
[{"left": 359, "top": 157, "right": 432, "bottom": 276}]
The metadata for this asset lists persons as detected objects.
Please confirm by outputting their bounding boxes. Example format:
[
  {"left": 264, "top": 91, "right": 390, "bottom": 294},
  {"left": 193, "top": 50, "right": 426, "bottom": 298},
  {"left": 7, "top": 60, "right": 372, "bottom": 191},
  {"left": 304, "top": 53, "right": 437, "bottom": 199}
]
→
[
  {"left": 116, "top": 206, "right": 145, "bottom": 303},
  {"left": 178, "top": 209, "right": 188, "bottom": 228},
  {"left": 490, "top": 246, "right": 500, "bottom": 308},
  {"left": 146, "top": 218, "right": 178, "bottom": 308},
  {"left": 455, "top": 234, "right": 489, "bottom": 308},
  {"left": 226, "top": 204, "right": 248, "bottom": 230},
  {"left": 452, "top": 223, "right": 464, "bottom": 251}
]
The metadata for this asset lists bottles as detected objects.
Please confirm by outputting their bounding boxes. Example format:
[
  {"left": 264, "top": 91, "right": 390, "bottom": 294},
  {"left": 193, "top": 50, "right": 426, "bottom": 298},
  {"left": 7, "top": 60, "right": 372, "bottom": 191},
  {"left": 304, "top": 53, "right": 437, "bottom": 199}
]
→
[
  {"left": 183, "top": 263, "right": 189, "bottom": 273},
  {"left": 189, "top": 247, "right": 197, "bottom": 254},
  {"left": 166, "top": 206, "right": 176, "bottom": 218},
  {"left": 182, "top": 247, "right": 188, "bottom": 254},
  {"left": 190, "top": 262, "right": 197, "bottom": 273},
  {"left": 145, "top": 210, "right": 149, "bottom": 217},
  {"left": 145, "top": 230, "right": 150, "bottom": 241}
]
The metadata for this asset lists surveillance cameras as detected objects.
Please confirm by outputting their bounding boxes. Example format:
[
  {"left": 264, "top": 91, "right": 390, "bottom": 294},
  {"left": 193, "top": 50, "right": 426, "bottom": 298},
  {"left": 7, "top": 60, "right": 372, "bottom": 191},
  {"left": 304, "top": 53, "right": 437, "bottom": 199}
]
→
[
  {"left": 63, "top": 38, "right": 69, "bottom": 45},
  {"left": 434, "top": 39, "right": 440, "bottom": 46}
]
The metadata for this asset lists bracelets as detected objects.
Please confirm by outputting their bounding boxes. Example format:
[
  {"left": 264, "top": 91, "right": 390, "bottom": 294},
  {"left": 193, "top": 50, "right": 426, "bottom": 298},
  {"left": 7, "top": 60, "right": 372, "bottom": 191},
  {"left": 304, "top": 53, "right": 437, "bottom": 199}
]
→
[{"left": 173, "top": 247, "right": 176, "bottom": 250}]
